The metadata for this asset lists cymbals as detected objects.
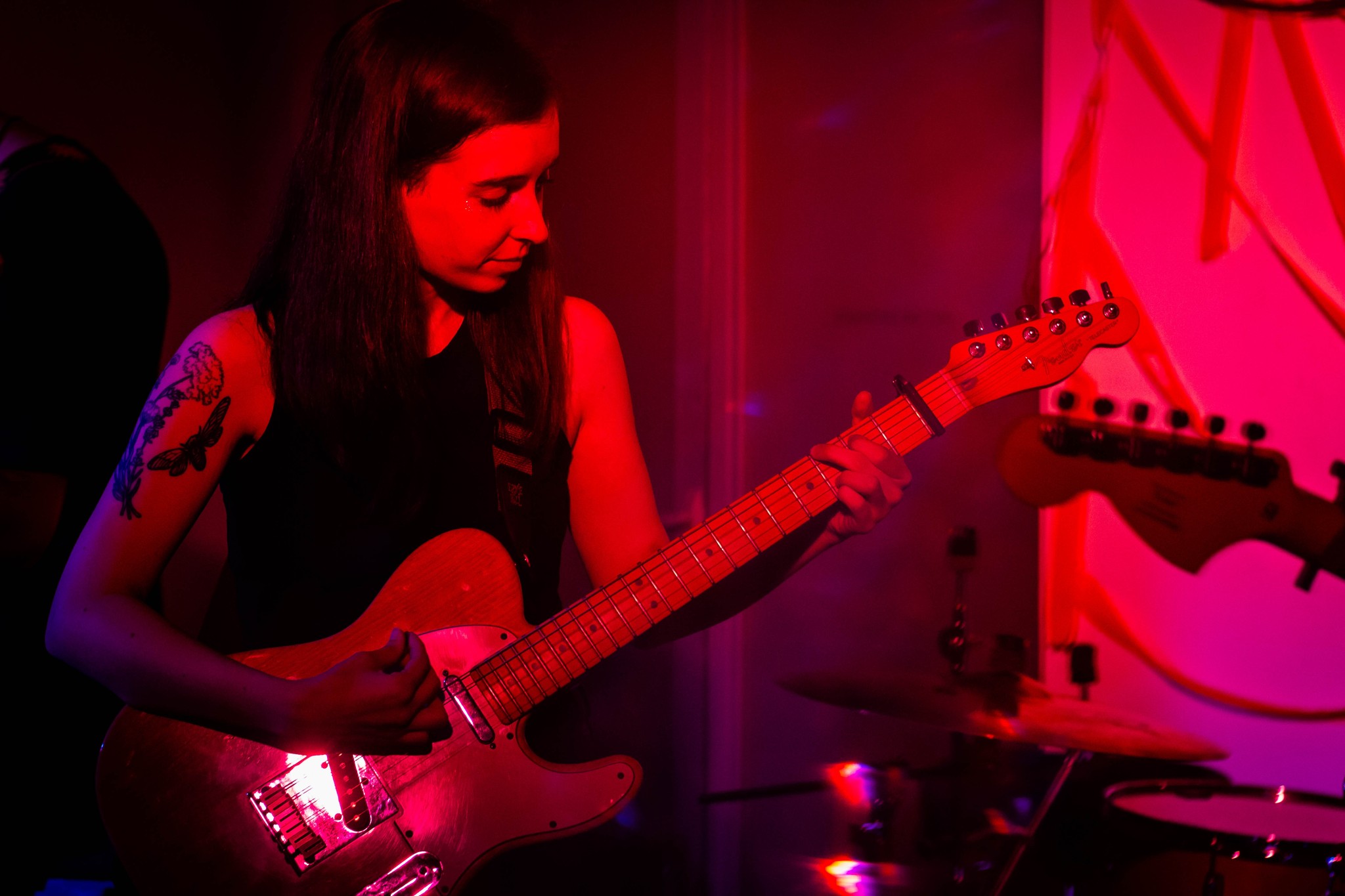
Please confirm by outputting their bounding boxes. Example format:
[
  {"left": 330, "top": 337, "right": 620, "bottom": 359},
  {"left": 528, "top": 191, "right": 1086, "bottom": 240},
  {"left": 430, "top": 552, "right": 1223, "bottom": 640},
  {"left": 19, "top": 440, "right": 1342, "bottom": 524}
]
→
[{"left": 769, "top": 668, "right": 1234, "bottom": 763}]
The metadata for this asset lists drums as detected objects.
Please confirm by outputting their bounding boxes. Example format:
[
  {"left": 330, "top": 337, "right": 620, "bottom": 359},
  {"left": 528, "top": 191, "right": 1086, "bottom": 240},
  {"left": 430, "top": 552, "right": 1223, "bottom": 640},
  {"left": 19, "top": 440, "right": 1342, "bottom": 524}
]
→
[{"left": 1096, "top": 776, "right": 1344, "bottom": 896}]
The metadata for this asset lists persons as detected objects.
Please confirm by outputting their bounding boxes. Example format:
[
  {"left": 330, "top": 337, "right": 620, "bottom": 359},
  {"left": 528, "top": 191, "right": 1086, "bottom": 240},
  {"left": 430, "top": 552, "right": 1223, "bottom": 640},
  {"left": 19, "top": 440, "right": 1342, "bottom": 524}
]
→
[
  {"left": 0, "top": 103, "right": 168, "bottom": 615},
  {"left": 44, "top": 0, "right": 913, "bottom": 896}
]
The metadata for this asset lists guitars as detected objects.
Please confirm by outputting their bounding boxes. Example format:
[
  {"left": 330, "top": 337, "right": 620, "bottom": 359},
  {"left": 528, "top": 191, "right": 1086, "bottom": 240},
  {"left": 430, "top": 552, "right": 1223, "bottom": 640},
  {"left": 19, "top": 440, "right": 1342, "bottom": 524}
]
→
[
  {"left": 99, "top": 280, "right": 1145, "bottom": 896},
  {"left": 998, "top": 386, "right": 1345, "bottom": 592}
]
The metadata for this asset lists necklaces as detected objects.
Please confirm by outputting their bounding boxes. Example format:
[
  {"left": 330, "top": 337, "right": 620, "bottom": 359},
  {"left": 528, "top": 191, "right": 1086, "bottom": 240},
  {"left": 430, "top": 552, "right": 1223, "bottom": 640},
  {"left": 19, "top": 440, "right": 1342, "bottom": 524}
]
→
[{"left": 0, "top": 112, "right": 20, "bottom": 141}]
